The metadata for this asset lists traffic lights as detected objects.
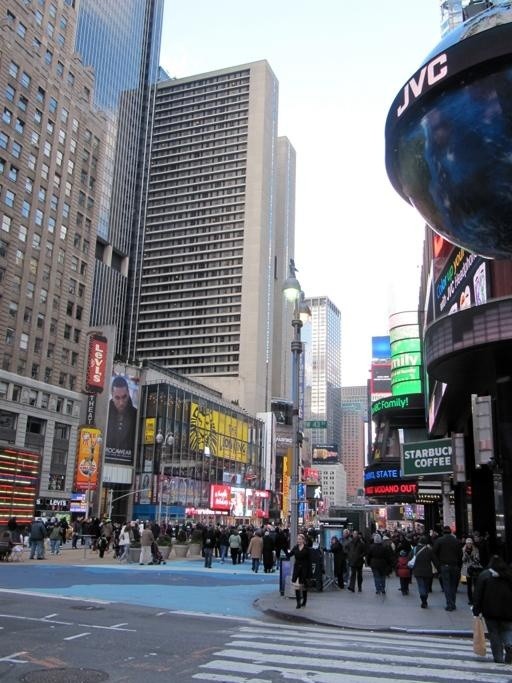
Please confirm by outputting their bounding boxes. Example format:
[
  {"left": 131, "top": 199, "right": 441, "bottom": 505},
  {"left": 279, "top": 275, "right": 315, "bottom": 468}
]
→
[
  {"left": 270, "top": 399, "right": 293, "bottom": 411},
  {"left": 305, "top": 483, "right": 323, "bottom": 501}
]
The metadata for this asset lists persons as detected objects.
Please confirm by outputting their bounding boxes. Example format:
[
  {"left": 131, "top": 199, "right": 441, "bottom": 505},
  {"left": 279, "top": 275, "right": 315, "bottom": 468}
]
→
[
  {"left": 106, "top": 376, "right": 137, "bottom": 460},
  {"left": 472, "top": 554, "right": 512, "bottom": 664},
  {"left": 283, "top": 497, "right": 512, "bottom": 612},
  {"left": 0, "top": 513, "right": 290, "bottom": 576}
]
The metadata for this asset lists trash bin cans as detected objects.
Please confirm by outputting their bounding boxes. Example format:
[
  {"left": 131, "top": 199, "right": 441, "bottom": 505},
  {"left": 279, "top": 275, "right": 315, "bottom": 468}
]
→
[{"left": 305, "top": 549, "right": 323, "bottom": 594}]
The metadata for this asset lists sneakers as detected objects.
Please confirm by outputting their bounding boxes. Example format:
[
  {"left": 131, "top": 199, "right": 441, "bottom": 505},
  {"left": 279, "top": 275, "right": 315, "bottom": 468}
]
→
[
  {"left": 446, "top": 606, "right": 452, "bottom": 611},
  {"left": 504, "top": 644, "right": 511, "bottom": 665},
  {"left": 452, "top": 606, "right": 456, "bottom": 610}
]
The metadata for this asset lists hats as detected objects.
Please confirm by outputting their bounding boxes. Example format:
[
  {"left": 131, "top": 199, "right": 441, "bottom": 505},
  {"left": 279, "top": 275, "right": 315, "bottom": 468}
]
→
[
  {"left": 374, "top": 534, "right": 381, "bottom": 544},
  {"left": 466, "top": 538, "right": 473, "bottom": 545},
  {"left": 400, "top": 550, "right": 405, "bottom": 556},
  {"left": 265, "top": 531, "right": 269, "bottom": 536}
]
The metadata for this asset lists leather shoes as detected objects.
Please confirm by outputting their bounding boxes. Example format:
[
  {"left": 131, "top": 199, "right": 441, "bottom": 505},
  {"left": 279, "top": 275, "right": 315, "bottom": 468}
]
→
[{"left": 348, "top": 586, "right": 354, "bottom": 592}]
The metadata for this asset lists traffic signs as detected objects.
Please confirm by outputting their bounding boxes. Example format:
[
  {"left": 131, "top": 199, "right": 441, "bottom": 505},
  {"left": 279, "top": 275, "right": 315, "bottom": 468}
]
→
[{"left": 301, "top": 419, "right": 329, "bottom": 429}]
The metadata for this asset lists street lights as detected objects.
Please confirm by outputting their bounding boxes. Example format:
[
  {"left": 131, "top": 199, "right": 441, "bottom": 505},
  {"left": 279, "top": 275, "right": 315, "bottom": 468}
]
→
[
  {"left": 82, "top": 429, "right": 102, "bottom": 520},
  {"left": 239, "top": 461, "right": 254, "bottom": 517},
  {"left": 155, "top": 428, "right": 174, "bottom": 527},
  {"left": 163, "top": 477, "right": 175, "bottom": 528},
  {"left": 277, "top": 254, "right": 313, "bottom": 565}
]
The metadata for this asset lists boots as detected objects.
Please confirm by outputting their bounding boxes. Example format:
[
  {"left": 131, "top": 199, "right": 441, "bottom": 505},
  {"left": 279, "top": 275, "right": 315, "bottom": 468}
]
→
[
  {"left": 295, "top": 590, "right": 301, "bottom": 609},
  {"left": 302, "top": 590, "right": 307, "bottom": 606}
]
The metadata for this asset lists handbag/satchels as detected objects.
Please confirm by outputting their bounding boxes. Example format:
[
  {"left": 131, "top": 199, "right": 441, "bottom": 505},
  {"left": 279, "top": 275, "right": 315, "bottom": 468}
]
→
[
  {"left": 407, "top": 555, "right": 416, "bottom": 569},
  {"left": 471, "top": 615, "right": 486, "bottom": 657}
]
